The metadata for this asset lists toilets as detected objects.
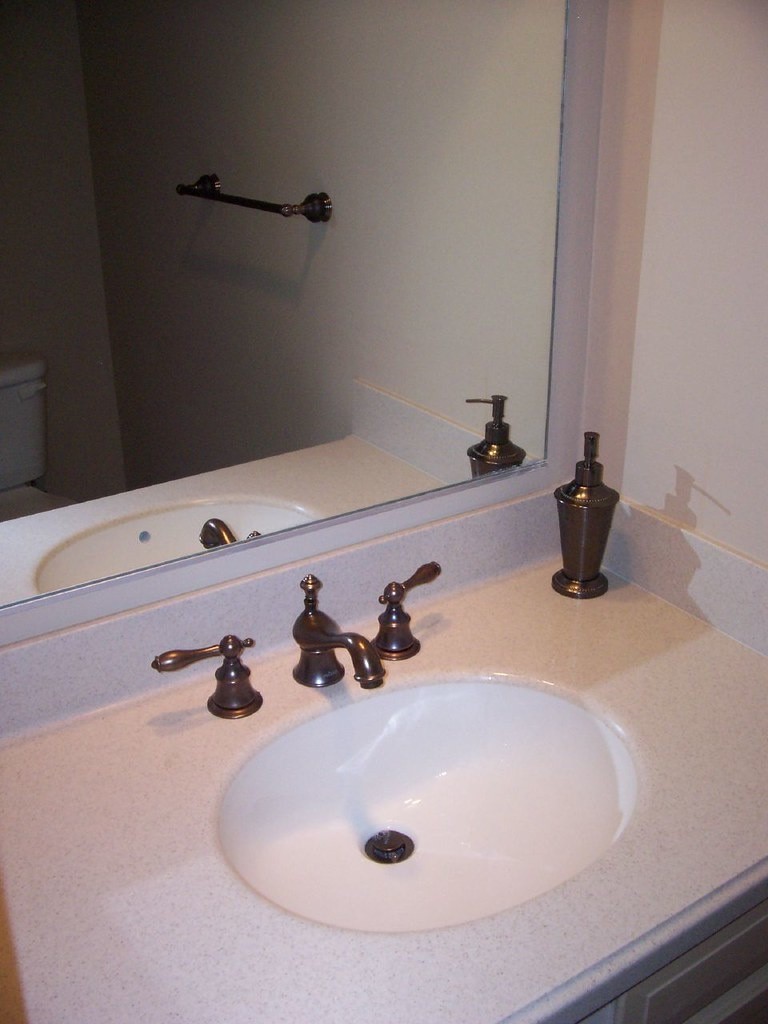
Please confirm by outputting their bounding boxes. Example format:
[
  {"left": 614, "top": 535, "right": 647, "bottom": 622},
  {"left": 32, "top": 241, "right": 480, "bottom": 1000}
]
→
[{"left": 0, "top": 353, "right": 79, "bottom": 521}]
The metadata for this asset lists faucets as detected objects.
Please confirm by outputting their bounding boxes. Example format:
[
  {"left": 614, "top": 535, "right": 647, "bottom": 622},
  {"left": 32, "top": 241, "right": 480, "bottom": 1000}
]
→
[
  {"left": 200, "top": 518, "right": 236, "bottom": 549},
  {"left": 292, "top": 573, "right": 386, "bottom": 690}
]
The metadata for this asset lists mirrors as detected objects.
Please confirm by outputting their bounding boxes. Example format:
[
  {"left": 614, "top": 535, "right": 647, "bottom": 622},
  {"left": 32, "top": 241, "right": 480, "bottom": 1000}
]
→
[{"left": 0, "top": 0, "right": 608, "bottom": 627}]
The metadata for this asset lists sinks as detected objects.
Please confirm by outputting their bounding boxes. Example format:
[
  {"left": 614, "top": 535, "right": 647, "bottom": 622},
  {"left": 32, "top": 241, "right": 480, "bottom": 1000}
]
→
[
  {"left": 33, "top": 494, "right": 320, "bottom": 594},
  {"left": 217, "top": 673, "right": 638, "bottom": 934}
]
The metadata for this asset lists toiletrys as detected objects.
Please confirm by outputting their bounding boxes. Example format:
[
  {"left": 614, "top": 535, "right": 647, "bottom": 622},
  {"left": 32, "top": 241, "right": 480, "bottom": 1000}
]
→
[
  {"left": 466, "top": 395, "right": 525, "bottom": 480},
  {"left": 550, "top": 432, "right": 620, "bottom": 599}
]
